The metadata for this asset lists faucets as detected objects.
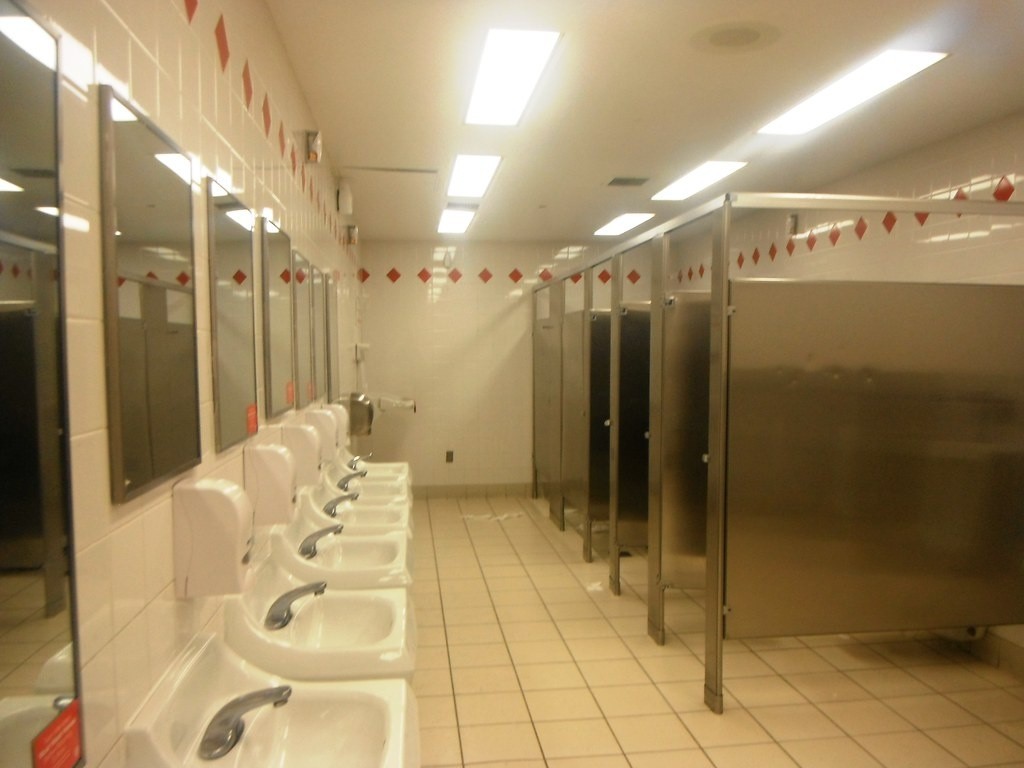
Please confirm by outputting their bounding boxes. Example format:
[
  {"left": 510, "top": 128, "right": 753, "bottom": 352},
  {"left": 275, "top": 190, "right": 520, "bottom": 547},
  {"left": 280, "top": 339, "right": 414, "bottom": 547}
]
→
[
  {"left": 322, "top": 492, "right": 360, "bottom": 518},
  {"left": 338, "top": 470, "right": 368, "bottom": 491},
  {"left": 264, "top": 582, "right": 327, "bottom": 630},
  {"left": 346, "top": 450, "right": 375, "bottom": 470},
  {"left": 54, "top": 696, "right": 75, "bottom": 711},
  {"left": 195, "top": 687, "right": 291, "bottom": 758},
  {"left": 299, "top": 523, "right": 344, "bottom": 561}
]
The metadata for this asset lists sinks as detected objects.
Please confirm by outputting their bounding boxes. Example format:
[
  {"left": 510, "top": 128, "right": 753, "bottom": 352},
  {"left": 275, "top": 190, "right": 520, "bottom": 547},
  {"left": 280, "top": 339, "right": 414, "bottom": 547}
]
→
[
  {"left": 270, "top": 506, "right": 414, "bottom": 586},
  {"left": 334, "top": 445, "right": 414, "bottom": 487},
  {"left": 127, "top": 629, "right": 420, "bottom": 767},
  {"left": 321, "top": 458, "right": 414, "bottom": 512},
  {"left": 224, "top": 552, "right": 419, "bottom": 677},
  {"left": 302, "top": 473, "right": 415, "bottom": 534},
  {"left": 37, "top": 641, "right": 76, "bottom": 693},
  {"left": 2, "top": 694, "right": 57, "bottom": 768}
]
